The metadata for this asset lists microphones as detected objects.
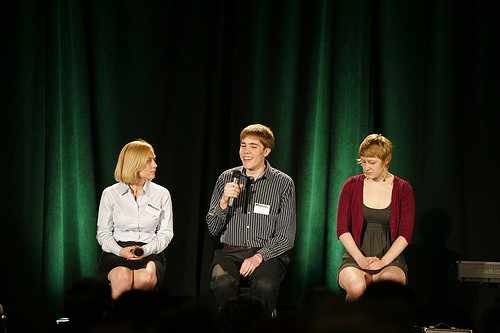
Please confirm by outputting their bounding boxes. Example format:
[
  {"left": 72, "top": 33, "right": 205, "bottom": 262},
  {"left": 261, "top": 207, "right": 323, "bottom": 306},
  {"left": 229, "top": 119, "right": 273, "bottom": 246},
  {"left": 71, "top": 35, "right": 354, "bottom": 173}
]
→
[
  {"left": 227, "top": 170, "right": 241, "bottom": 207},
  {"left": 131, "top": 248, "right": 143, "bottom": 256}
]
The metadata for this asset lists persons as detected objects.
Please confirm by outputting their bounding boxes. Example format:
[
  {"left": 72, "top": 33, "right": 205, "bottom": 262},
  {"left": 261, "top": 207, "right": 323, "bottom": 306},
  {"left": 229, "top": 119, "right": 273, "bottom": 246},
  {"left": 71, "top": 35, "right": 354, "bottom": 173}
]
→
[
  {"left": 0, "top": 254, "right": 500, "bottom": 333},
  {"left": 206, "top": 124, "right": 296, "bottom": 279},
  {"left": 95, "top": 140, "right": 173, "bottom": 298},
  {"left": 337, "top": 134, "right": 415, "bottom": 301}
]
